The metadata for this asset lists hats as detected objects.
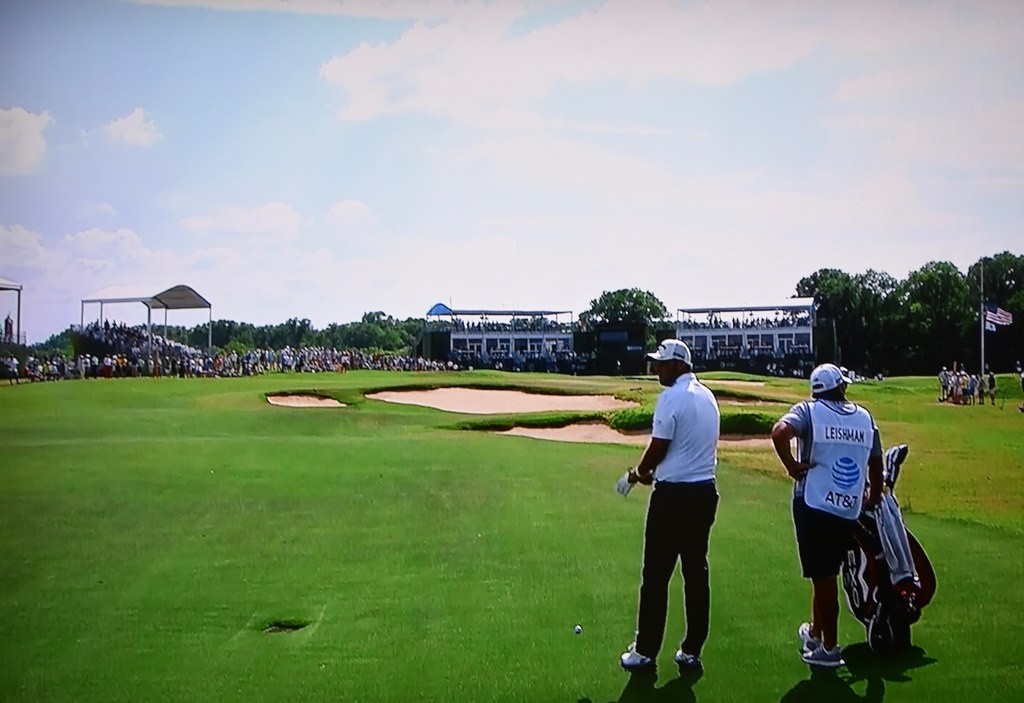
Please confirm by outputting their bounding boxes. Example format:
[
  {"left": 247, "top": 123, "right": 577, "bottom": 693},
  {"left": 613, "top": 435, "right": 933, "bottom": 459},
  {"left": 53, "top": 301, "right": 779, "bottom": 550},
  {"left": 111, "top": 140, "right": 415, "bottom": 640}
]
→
[
  {"left": 810, "top": 363, "right": 853, "bottom": 392},
  {"left": 644, "top": 339, "right": 693, "bottom": 369}
]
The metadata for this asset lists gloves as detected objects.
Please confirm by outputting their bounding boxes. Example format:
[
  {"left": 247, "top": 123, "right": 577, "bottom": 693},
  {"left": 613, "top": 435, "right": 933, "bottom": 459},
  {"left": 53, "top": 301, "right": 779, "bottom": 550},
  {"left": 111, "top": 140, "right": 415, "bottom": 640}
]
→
[{"left": 616, "top": 467, "right": 640, "bottom": 496}]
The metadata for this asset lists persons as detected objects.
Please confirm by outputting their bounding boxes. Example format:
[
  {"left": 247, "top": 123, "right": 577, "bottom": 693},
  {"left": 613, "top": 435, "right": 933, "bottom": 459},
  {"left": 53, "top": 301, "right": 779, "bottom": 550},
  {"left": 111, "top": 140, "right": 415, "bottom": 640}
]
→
[
  {"left": 937, "top": 366, "right": 996, "bottom": 407},
  {"left": 769, "top": 363, "right": 885, "bottom": 667},
  {"left": 615, "top": 339, "right": 722, "bottom": 672},
  {"left": 0, "top": 317, "right": 459, "bottom": 385}
]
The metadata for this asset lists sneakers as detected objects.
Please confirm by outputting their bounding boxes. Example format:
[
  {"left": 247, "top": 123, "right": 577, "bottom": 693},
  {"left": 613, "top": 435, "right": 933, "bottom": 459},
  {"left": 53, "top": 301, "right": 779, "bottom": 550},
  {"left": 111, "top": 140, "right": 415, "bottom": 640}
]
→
[
  {"left": 802, "top": 642, "right": 845, "bottom": 666},
  {"left": 675, "top": 649, "right": 702, "bottom": 667},
  {"left": 798, "top": 621, "right": 821, "bottom": 652},
  {"left": 620, "top": 643, "right": 654, "bottom": 667}
]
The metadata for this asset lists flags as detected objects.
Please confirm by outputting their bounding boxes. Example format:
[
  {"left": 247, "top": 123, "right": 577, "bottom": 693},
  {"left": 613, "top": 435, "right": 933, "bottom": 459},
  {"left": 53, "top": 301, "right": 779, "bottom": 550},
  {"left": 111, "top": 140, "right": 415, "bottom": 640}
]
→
[{"left": 984, "top": 305, "right": 1013, "bottom": 332}]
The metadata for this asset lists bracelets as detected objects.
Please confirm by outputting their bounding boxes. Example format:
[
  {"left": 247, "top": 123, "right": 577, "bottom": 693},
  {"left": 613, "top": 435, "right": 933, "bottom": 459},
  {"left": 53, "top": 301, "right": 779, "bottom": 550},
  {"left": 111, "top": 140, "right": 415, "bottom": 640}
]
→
[{"left": 634, "top": 466, "right": 644, "bottom": 480}]
